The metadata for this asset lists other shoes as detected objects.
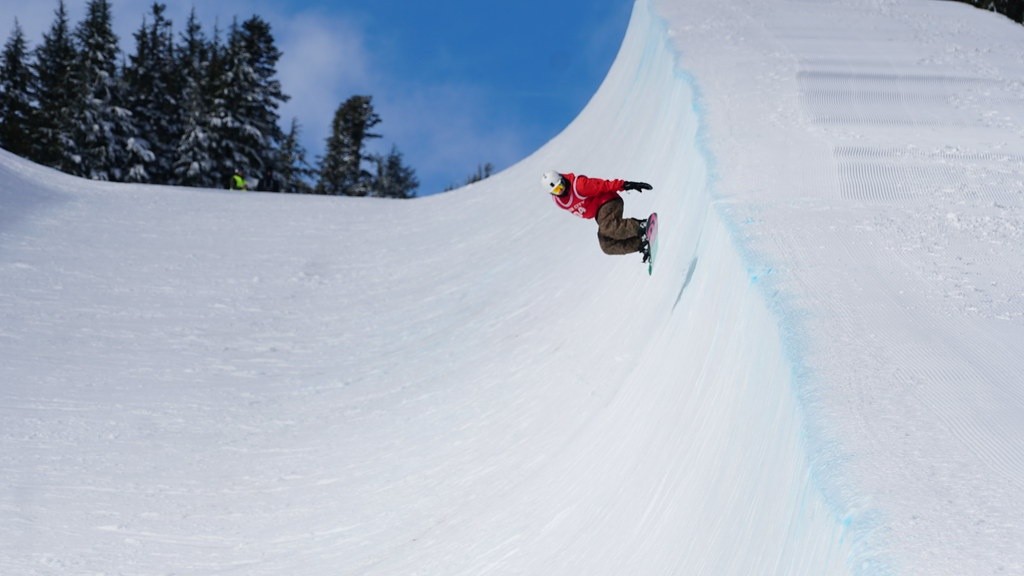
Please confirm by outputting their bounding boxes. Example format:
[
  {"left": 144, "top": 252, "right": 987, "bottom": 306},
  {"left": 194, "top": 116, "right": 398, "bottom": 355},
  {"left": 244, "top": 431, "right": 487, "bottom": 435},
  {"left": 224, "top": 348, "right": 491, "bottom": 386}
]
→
[
  {"left": 638, "top": 218, "right": 648, "bottom": 238},
  {"left": 638, "top": 242, "right": 649, "bottom": 262}
]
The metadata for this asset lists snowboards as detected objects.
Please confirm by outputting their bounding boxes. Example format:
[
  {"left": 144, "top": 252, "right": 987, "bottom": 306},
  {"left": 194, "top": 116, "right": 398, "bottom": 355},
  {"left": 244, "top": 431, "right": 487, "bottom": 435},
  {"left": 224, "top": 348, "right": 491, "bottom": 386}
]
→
[{"left": 645, "top": 213, "right": 659, "bottom": 275}]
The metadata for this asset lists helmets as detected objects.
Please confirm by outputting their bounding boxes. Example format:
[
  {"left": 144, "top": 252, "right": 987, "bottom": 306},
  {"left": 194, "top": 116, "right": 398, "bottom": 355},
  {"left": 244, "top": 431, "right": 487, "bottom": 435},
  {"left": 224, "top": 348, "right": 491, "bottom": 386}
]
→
[{"left": 542, "top": 170, "right": 561, "bottom": 192}]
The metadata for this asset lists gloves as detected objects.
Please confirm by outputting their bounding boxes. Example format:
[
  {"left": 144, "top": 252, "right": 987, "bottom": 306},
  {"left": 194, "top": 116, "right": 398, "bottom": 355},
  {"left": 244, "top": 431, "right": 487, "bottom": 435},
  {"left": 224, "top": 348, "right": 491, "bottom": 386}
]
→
[{"left": 623, "top": 181, "right": 653, "bottom": 193}]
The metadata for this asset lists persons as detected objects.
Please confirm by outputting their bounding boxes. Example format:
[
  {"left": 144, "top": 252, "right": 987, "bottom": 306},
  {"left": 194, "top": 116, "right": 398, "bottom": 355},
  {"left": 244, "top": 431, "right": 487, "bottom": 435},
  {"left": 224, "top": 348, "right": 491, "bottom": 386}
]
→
[
  {"left": 540, "top": 170, "right": 653, "bottom": 263},
  {"left": 230, "top": 167, "right": 248, "bottom": 191}
]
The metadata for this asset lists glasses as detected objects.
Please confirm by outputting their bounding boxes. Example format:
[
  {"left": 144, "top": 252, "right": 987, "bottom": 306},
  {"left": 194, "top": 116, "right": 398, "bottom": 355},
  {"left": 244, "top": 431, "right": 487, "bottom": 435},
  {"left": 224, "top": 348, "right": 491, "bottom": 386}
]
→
[{"left": 552, "top": 183, "right": 563, "bottom": 193}]
